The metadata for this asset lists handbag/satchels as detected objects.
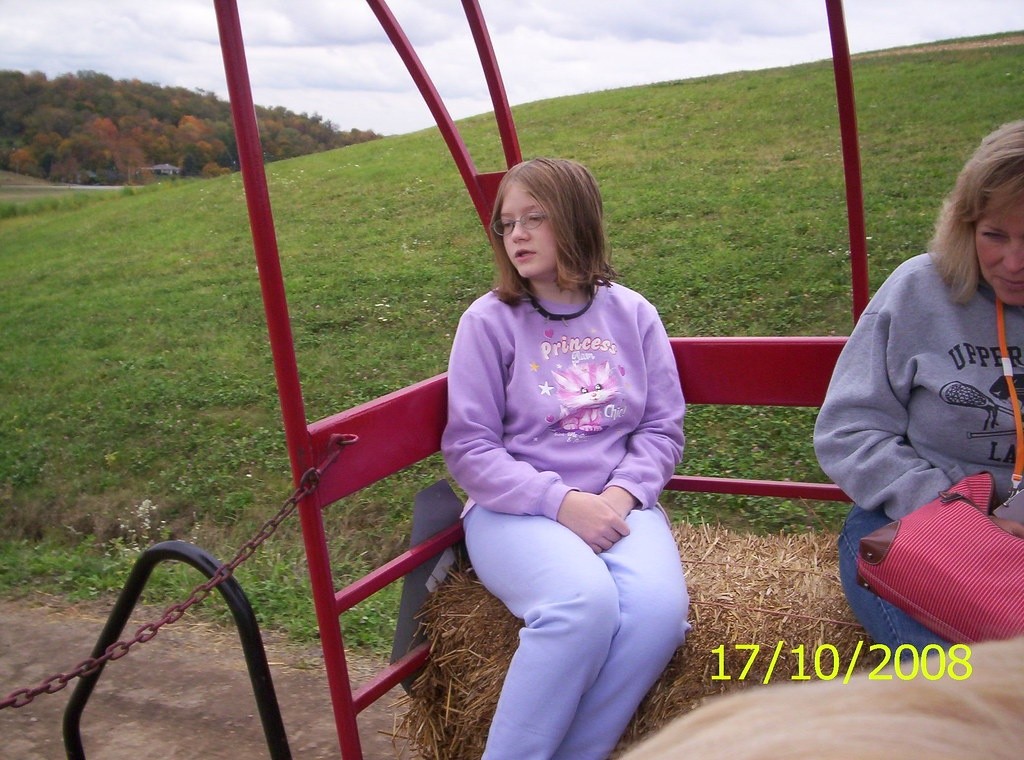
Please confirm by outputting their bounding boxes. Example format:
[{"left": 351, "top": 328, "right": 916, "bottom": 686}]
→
[{"left": 855, "top": 471, "right": 1024, "bottom": 645}]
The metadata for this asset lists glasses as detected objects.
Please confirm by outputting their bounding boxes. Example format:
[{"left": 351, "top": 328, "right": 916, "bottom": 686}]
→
[{"left": 491, "top": 211, "right": 548, "bottom": 236}]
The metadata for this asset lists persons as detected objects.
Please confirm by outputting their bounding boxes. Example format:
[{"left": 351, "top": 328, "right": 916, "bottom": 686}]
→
[
  {"left": 441, "top": 157, "right": 688, "bottom": 760},
  {"left": 813, "top": 119, "right": 1024, "bottom": 665}
]
